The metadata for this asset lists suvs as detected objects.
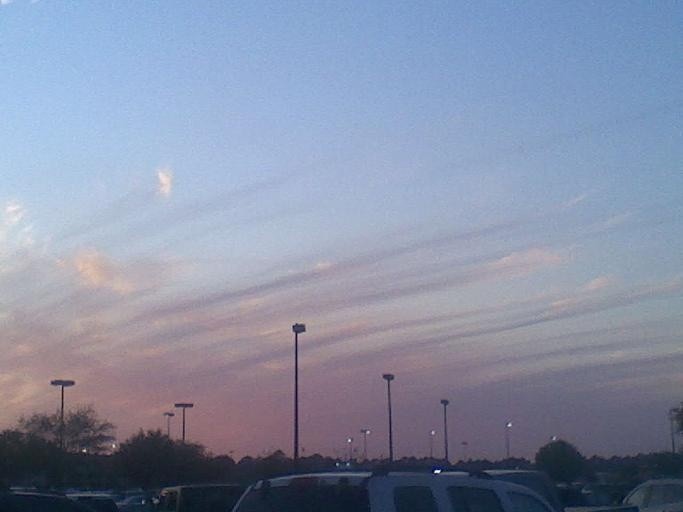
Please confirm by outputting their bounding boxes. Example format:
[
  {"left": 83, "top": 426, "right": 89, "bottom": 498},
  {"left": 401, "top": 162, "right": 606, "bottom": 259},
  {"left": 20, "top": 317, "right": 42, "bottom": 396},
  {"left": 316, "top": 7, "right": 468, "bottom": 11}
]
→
[
  {"left": 439, "top": 470, "right": 565, "bottom": 512},
  {"left": 232, "top": 466, "right": 555, "bottom": 512}
]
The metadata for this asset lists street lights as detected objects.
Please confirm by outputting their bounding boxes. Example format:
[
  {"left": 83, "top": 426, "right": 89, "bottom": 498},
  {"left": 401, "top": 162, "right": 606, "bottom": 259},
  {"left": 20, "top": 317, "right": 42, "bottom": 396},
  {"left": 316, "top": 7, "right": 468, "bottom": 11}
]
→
[
  {"left": 361, "top": 429, "right": 370, "bottom": 456},
  {"left": 293, "top": 321, "right": 306, "bottom": 463},
  {"left": 505, "top": 422, "right": 513, "bottom": 457},
  {"left": 51, "top": 379, "right": 75, "bottom": 421},
  {"left": 441, "top": 399, "right": 449, "bottom": 464},
  {"left": 164, "top": 402, "right": 194, "bottom": 444},
  {"left": 383, "top": 374, "right": 394, "bottom": 465},
  {"left": 429, "top": 430, "right": 436, "bottom": 457},
  {"left": 346, "top": 436, "right": 353, "bottom": 460}
]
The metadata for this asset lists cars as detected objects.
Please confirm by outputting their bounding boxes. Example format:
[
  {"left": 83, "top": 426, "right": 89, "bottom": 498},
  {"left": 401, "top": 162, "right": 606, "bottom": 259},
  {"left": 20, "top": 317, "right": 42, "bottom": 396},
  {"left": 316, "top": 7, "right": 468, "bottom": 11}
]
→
[
  {"left": 0, "top": 485, "right": 247, "bottom": 512},
  {"left": 617, "top": 478, "right": 683, "bottom": 512}
]
